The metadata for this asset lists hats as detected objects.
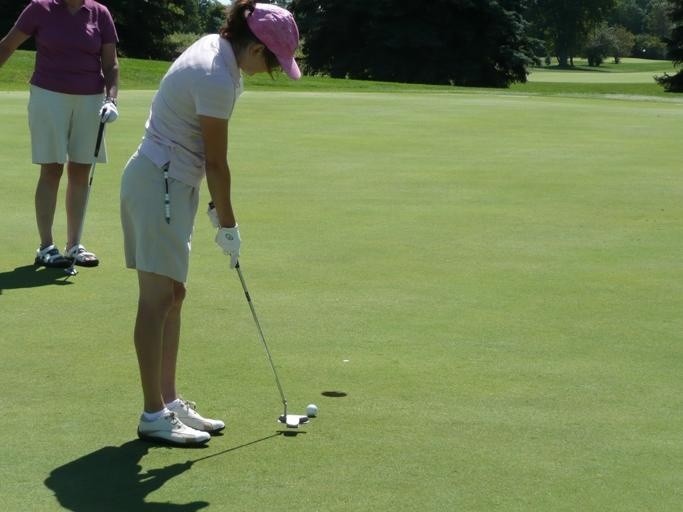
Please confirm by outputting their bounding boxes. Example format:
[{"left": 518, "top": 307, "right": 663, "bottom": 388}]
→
[{"left": 246, "top": 3, "right": 302, "bottom": 81}]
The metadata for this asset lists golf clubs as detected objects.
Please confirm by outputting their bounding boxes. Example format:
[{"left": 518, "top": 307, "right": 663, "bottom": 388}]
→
[
  {"left": 64, "top": 108, "right": 108, "bottom": 276},
  {"left": 235, "top": 258, "right": 310, "bottom": 428}
]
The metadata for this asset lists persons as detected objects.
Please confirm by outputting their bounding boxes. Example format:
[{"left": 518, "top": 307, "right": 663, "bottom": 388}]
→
[
  {"left": 119, "top": 1, "right": 302, "bottom": 447},
  {"left": 0, "top": 0, "right": 120, "bottom": 268}
]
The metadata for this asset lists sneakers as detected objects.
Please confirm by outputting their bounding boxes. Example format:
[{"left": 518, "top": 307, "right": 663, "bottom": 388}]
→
[
  {"left": 137, "top": 410, "right": 210, "bottom": 446},
  {"left": 170, "top": 401, "right": 225, "bottom": 433}
]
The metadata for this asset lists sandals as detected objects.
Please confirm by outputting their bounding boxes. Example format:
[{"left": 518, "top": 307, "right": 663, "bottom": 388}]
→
[
  {"left": 64, "top": 244, "right": 99, "bottom": 267},
  {"left": 35, "top": 245, "right": 70, "bottom": 269}
]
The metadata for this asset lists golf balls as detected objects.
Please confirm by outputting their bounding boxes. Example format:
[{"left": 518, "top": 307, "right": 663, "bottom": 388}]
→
[{"left": 307, "top": 404, "right": 318, "bottom": 416}]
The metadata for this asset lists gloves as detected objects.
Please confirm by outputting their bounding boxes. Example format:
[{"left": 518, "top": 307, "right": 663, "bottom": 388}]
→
[
  {"left": 99, "top": 103, "right": 118, "bottom": 124},
  {"left": 215, "top": 222, "right": 241, "bottom": 268},
  {"left": 207, "top": 205, "right": 220, "bottom": 228}
]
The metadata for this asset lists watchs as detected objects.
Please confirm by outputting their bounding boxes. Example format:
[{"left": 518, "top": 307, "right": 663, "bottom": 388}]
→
[{"left": 106, "top": 97, "right": 117, "bottom": 106}]
